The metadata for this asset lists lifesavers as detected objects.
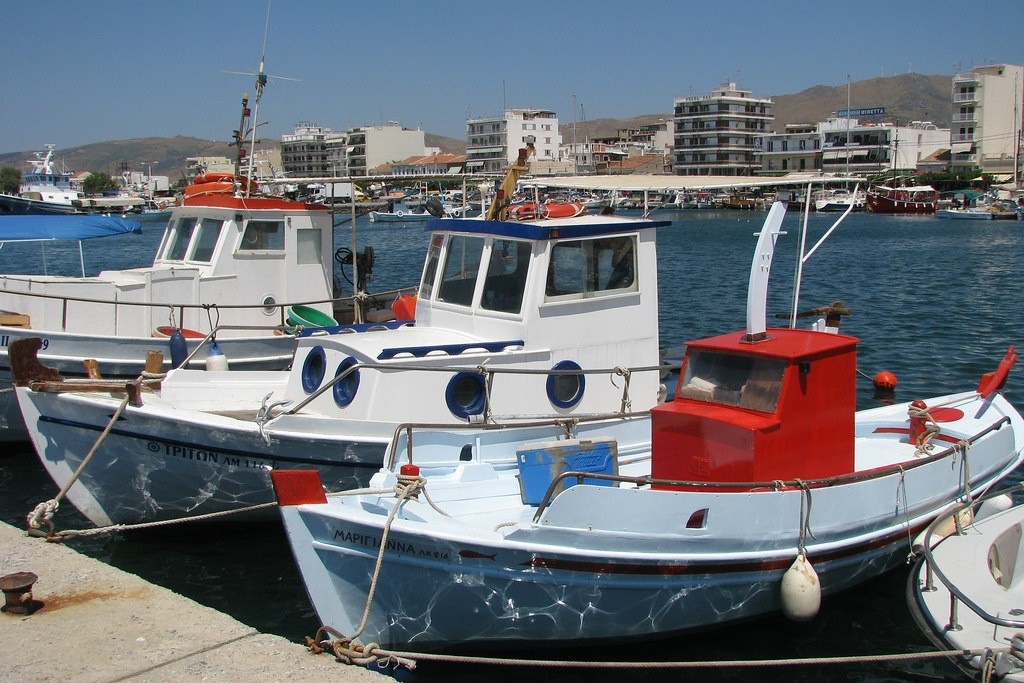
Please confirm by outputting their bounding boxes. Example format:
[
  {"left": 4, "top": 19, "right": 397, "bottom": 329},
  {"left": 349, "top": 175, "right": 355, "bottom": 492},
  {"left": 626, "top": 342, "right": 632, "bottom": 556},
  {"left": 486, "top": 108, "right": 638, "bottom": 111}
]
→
[
  {"left": 194, "top": 172, "right": 259, "bottom": 191},
  {"left": 503, "top": 202, "right": 586, "bottom": 220}
]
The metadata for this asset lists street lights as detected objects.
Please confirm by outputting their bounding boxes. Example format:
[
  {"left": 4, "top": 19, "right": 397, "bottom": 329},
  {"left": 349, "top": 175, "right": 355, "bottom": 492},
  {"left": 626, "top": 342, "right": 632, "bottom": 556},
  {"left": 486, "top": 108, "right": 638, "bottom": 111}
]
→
[{"left": 140, "top": 161, "right": 159, "bottom": 199}]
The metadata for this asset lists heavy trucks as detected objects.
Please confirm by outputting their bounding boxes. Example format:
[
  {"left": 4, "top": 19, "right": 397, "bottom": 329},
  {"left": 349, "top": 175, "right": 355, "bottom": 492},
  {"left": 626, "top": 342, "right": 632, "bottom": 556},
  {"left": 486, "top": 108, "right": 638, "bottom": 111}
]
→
[{"left": 306, "top": 182, "right": 351, "bottom": 204}]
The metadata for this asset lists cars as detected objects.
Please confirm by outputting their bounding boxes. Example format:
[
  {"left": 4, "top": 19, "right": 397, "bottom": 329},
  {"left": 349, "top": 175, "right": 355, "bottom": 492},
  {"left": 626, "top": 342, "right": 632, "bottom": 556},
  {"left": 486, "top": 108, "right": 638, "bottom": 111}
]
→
[
  {"left": 453, "top": 193, "right": 463, "bottom": 203},
  {"left": 371, "top": 191, "right": 384, "bottom": 200}
]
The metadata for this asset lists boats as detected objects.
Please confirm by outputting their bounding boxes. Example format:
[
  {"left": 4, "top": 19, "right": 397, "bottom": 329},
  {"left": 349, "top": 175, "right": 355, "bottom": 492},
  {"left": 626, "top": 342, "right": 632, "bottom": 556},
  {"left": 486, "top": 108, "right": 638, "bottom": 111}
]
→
[
  {"left": 0, "top": 142, "right": 88, "bottom": 215},
  {"left": 0, "top": 0, "right": 536, "bottom": 379},
  {"left": 903, "top": 492, "right": 1024, "bottom": 683},
  {"left": 270, "top": 179, "right": 1023, "bottom": 664},
  {"left": 367, "top": 179, "right": 1024, "bottom": 223},
  {"left": 12, "top": 204, "right": 676, "bottom": 539}
]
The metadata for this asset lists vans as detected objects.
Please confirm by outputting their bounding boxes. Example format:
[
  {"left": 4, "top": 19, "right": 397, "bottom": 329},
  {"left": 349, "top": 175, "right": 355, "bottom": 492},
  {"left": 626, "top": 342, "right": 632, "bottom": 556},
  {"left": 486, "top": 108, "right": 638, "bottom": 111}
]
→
[
  {"left": 466, "top": 190, "right": 482, "bottom": 201},
  {"left": 438, "top": 190, "right": 461, "bottom": 202}
]
